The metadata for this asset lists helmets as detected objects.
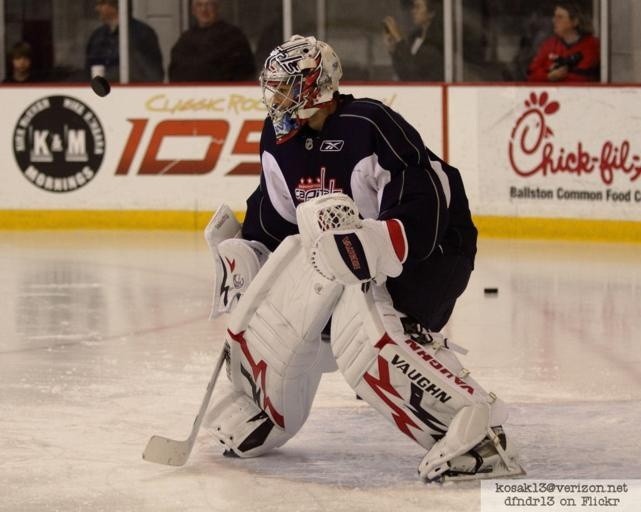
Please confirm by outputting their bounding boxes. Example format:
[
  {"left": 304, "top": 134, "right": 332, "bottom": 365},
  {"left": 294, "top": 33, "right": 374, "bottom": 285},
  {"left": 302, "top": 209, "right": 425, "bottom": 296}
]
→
[{"left": 259, "top": 33, "right": 343, "bottom": 145}]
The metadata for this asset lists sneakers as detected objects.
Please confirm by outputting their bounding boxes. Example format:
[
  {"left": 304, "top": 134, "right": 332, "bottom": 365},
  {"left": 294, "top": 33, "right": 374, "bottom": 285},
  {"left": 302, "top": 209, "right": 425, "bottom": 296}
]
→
[{"left": 448, "top": 424, "right": 507, "bottom": 471}]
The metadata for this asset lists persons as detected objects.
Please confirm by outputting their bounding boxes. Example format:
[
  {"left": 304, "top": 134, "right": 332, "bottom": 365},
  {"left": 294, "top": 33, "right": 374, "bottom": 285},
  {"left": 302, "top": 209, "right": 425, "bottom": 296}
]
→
[
  {"left": 203, "top": 33, "right": 529, "bottom": 485},
  {"left": 1, "top": 0, "right": 604, "bottom": 87}
]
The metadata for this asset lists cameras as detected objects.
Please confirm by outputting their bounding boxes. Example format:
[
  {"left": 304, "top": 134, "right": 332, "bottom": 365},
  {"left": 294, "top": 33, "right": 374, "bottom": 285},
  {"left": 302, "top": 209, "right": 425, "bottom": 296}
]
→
[{"left": 552, "top": 49, "right": 583, "bottom": 72}]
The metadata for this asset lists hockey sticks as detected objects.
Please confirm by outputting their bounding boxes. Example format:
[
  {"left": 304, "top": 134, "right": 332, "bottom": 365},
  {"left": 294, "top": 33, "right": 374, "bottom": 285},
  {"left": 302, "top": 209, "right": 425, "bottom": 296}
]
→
[{"left": 142, "top": 348, "right": 225, "bottom": 466}]
